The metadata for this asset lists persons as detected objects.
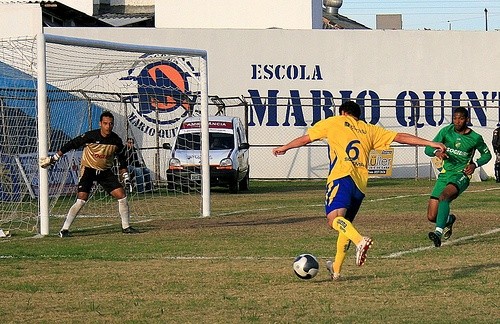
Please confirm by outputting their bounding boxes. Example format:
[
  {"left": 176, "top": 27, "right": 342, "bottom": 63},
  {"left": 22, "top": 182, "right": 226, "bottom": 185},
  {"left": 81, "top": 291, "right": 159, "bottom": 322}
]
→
[
  {"left": 39, "top": 111, "right": 139, "bottom": 239},
  {"left": 492, "top": 123, "right": 500, "bottom": 183},
  {"left": 114, "top": 138, "right": 143, "bottom": 191},
  {"left": 425, "top": 106, "right": 492, "bottom": 248},
  {"left": 272, "top": 101, "right": 446, "bottom": 281}
]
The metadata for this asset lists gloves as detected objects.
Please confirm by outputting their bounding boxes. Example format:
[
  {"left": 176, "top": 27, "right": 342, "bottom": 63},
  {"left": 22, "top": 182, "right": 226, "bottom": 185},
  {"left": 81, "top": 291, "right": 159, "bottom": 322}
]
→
[
  {"left": 122, "top": 172, "right": 131, "bottom": 187},
  {"left": 39, "top": 156, "right": 56, "bottom": 171}
]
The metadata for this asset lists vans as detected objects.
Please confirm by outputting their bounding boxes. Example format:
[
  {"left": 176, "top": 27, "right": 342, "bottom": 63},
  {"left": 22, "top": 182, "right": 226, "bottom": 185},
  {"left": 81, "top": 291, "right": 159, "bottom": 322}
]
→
[{"left": 162, "top": 115, "right": 251, "bottom": 193}]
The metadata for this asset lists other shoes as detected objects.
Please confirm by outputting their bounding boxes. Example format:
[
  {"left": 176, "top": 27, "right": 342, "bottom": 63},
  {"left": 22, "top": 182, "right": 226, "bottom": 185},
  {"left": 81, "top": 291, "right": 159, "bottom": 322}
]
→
[
  {"left": 355, "top": 236, "right": 374, "bottom": 266},
  {"left": 57, "top": 229, "right": 73, "bottom": 239},
  {"left": 121, "top": 226, "right": 140, "bottom": 234},
  {"left": 429, "top": 231, "right": 441, "bottom": 247},
  {"left": 326, "top": 261, "right": 341, "bottom": 281},
  {"left": 443, "top": 214, "right": 456, "bottom": 240}
]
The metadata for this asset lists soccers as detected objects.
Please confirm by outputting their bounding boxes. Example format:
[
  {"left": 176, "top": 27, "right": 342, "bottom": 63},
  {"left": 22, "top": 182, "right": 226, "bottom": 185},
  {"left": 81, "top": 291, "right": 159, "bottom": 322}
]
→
[{"left": 292, "top": 253, "right": 319, "bottom": 280}]
